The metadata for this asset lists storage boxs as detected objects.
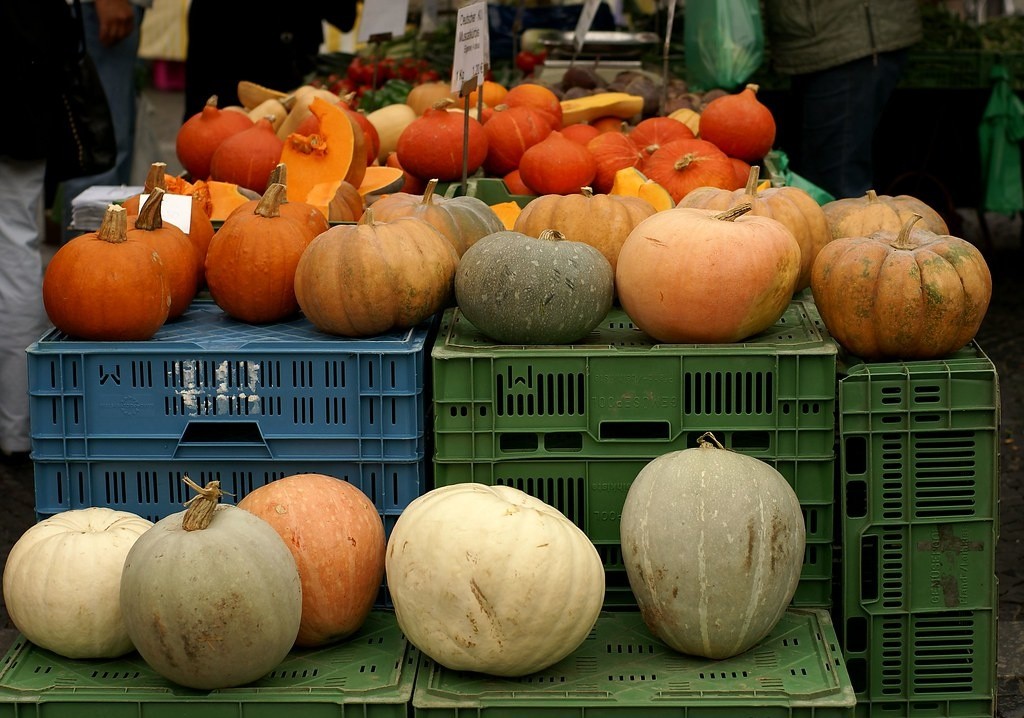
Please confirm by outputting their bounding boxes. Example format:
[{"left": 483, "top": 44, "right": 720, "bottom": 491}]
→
[
  {"left": 24, "top": 304, "right": 441, "bottom": 458},
  {"left": 0, "top": 609, "right": 420, "bottom": 718},
  {"left": 835, "top": 336, "right": 1001, "bottom": 717},
  {"left": 411, "top": 610, "right": 856, "bottom": 718},
  {"left": 36, "top": 457, "right": 433, "bottom": 613},
  {"left": 113, "top": 169, "right": 466, "bottom": 302},
  {"left": 463, "top": 178, "right": 788, "bottom": 208},
  {"left": 433, "top": 457, "right": 832, "bottom": 607},
  {"left": 432, "top": 285, "right": 837, "bottom": 459}
]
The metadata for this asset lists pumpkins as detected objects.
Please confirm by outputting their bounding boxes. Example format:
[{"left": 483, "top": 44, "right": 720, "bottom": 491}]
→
[
  {"left": 384, "top": 482, "right": 606, "bottom": 676},
  {"left": 235, "top": 473, "right": 386, "bottom": 647},
  {"left": 120, "top": 478, "right": 302, "bottom": 689},
  {"left": 620, "top": 442, "right": 805, "bottom": 659},
  {"left": 42, "top": 82, "right": 991, "bottom": 362},
  {"left": 2, "top": 506, "right": 160, "bottom": 658}
]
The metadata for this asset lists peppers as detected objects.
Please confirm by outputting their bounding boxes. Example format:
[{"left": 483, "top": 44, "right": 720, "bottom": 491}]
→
[{"left": 307, "top": 54, "right": 438, "bottom": 116}]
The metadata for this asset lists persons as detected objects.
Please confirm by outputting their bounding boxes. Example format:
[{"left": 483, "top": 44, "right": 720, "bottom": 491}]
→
[
  {"left": 0, "top": 0, "right": 364, "bottom": 470},
  {"left": 765, "top": 0, "right": 924, "bottom": 199}
]
